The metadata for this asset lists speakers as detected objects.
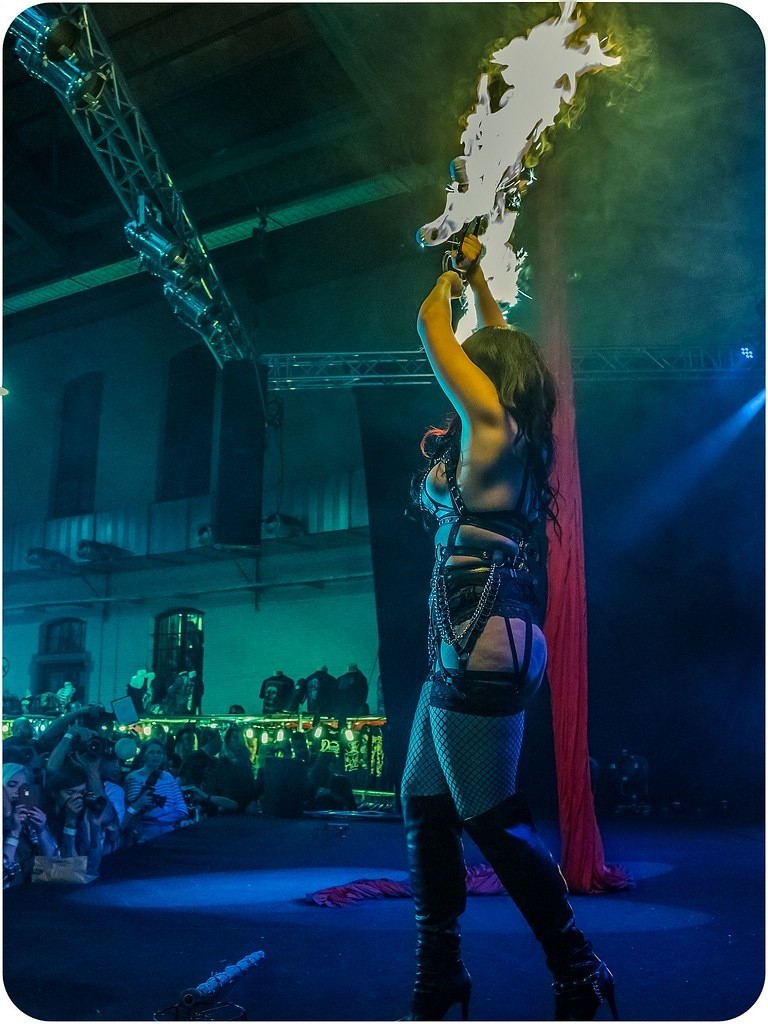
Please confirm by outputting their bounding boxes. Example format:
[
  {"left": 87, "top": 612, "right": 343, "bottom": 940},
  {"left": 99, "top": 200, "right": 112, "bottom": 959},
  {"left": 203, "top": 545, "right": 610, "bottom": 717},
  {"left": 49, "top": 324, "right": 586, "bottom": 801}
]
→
[
  {"left": 208, "top": 356, "right": 270, "bottom": 556},
  {"left": 257, "top": 758, "right": 303, "bottom": 820}
]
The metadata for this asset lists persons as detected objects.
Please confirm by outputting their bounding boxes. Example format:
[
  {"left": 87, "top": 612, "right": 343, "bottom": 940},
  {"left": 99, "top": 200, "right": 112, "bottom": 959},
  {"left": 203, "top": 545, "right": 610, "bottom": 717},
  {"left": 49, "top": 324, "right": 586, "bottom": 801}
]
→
[
  {"left": 3, "top": 624, "right": 385, "bottom": 924},
  {"left": 396, "top": 235, "right": 632, "bottom": 1018}
]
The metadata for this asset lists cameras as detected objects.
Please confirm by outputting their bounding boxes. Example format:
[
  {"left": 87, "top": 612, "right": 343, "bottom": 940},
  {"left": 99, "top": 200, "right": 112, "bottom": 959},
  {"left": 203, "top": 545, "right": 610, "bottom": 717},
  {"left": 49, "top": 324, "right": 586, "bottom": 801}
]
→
[
  {"left": 80, "top": 788, "right": 108, "bottom": 815},
  {"left": 183, "top": 790, "right": 196, "bottom": 801},
  {"left": 12, "top": 785, "right": 36, "bottom": 832},
  {"left": 72, "top": 734, "right": 120, "bottom": 761},
  {"left": 140, "top": 786, "right": 167, "bottom": 810},
  {"left": 79, "top": 711, "right": 113, "bottom": 735}
]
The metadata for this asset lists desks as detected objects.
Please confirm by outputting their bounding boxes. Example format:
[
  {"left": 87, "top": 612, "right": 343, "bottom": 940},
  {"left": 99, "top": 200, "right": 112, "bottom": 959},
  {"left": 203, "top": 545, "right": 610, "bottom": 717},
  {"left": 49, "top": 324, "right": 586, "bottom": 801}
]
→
[{"left": 2, "top": 711, "right": 387, "bottom": 790}]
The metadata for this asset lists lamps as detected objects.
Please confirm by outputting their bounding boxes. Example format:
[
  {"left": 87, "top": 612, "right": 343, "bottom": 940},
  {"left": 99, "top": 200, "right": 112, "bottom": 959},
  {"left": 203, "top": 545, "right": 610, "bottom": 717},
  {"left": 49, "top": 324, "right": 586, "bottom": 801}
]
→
[
  {"left": 124, "top": 195, "right": 182, "bottom": 267},
  {"left": 177, "top": 307, "right": 229, "bottom": 344},
  {"left": 138, "top": 245, "right": 200, "bottom": 290},
  {"left": 609, "top": 747, "right": 652, "bottom": 815},
  {"left": 8, "top": 5, "right": 80, "bottom": 63},
  {"left": 162, "top": 280, "right": 219, "bottom": 327},
  {"left": 14, "top": 38, "right": 106, "bottom": 112}
]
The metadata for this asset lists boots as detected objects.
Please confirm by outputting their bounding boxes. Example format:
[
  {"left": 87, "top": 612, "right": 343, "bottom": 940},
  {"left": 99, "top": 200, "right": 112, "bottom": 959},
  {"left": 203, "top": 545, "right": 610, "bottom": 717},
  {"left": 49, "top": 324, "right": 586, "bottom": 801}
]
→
[
  {"left": 462, "top": 791, "right": 619, "bottom": 1021},
  {"left": 392, "top": 794, "right": 474, "bottom": 1020}
]
